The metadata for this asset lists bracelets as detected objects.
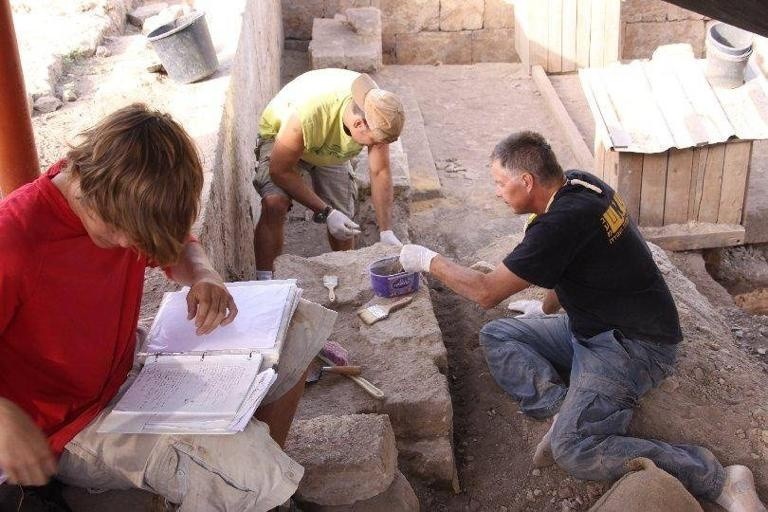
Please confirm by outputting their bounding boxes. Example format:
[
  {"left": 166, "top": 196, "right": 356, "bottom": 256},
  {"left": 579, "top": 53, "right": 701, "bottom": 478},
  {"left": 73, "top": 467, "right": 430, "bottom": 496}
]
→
[{"left": 315, "top": 205, "right": 334, "bottom": 224}]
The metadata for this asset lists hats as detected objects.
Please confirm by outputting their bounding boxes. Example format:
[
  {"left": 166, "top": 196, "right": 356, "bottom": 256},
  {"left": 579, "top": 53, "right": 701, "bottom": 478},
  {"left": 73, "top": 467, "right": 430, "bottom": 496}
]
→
[{"left": 351, "top": 72, "right": 406, "bottom": 145}]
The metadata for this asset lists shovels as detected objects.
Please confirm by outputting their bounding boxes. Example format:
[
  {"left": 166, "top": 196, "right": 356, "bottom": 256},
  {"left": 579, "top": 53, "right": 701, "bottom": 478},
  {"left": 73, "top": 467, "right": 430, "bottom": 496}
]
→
[{"left": 304, "top": 363, "right": 362, "bottom": 385}]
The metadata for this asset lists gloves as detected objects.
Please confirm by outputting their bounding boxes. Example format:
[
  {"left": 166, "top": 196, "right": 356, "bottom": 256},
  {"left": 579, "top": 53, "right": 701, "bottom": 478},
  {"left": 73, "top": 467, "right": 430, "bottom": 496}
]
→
[
  {"left": 399, "top": 243, "right": 439, "bottom": 274},
  {"left": 325, "top": 209, "right": 362, "bottom": 241},
  {"left": 379, "top": 229, "right": 402, "bottom": 248},
  {"left": 507, "top": 298, "right": 548, "bottom": 320}
]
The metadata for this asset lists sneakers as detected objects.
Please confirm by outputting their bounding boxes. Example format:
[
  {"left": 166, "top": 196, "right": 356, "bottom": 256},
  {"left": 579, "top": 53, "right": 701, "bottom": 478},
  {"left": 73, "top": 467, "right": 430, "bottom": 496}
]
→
[
  {"left": 532, "top": 412, "right": 564, "bottom": 468},
  {"left": 716, "top": 464, "right": 766, "bottom": 511}
]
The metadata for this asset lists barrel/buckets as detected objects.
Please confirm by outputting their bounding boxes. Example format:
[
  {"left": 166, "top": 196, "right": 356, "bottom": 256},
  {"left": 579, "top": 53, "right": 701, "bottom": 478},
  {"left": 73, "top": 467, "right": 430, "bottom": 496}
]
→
[
  {"left": 705, "top": 22, "right": 754, "bottom": 90},
  {"left": 146, "top": 11, "right": 219, "bottom": 85}
]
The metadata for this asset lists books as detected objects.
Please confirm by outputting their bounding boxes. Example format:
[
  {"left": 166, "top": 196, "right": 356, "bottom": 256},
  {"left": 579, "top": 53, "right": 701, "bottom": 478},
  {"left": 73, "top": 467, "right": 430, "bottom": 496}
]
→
[{"left": 96, "top": 277, "right": 306, "bottom": 434}]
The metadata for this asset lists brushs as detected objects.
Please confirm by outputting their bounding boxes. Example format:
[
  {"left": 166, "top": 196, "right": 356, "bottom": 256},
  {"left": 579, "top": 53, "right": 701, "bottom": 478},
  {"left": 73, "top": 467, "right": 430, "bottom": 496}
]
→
[
  {"left": 356, "top": 296, "right": 412, "bottom": 326},
  {"left": 323, "top": 275, "right": 338, "bottom": 303},
  {"left": 316, "top": 340, "right": 385, "bottom": 401}
]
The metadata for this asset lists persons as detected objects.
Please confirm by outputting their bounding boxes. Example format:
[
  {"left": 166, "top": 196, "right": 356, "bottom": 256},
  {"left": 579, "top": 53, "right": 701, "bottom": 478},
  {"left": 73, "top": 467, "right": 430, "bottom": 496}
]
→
[
  {"left": 1, "top": 100, "right": 338, "bottom": 510},
  {"left": 239, "top": 63, "right": 412, "bottom": 283},
  {"left": 394, "top": 127, "right": 768, "bottom": 512}
]
